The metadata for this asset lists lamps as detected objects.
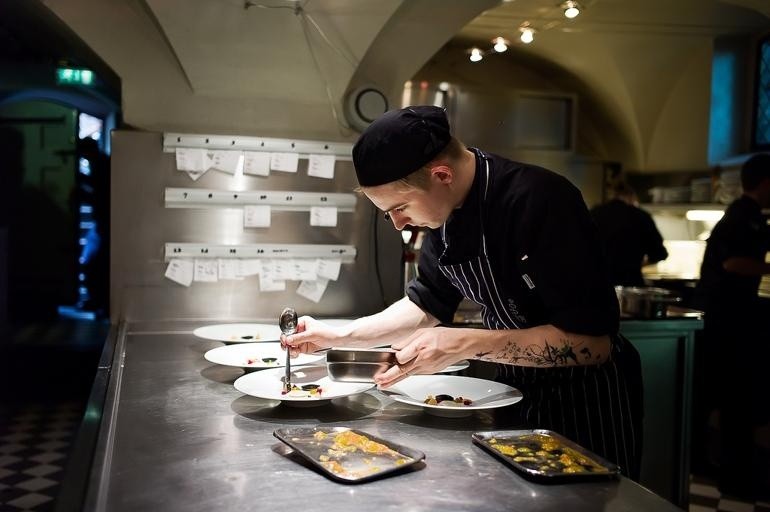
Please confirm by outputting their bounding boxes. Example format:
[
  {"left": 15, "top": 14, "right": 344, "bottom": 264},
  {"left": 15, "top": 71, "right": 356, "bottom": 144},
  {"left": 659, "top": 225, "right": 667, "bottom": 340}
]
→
[
  {"left": 470, "top": 48, "right": 483, "bottom": 62},
  {"left": 494, "top": 36, "right": 508, "bottom": 55},
  {"left": 517, "top": 25, "right": 537, "bottom": 44},
  {"left": 561, "top": 0, "right": 579, "bottom": 19}
]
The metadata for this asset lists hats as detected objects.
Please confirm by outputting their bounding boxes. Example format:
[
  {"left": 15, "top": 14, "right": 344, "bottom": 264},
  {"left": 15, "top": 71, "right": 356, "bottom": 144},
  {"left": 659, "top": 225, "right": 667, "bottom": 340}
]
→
[{"left": 352, "top": 106, "right": 450, "bottom": 187}]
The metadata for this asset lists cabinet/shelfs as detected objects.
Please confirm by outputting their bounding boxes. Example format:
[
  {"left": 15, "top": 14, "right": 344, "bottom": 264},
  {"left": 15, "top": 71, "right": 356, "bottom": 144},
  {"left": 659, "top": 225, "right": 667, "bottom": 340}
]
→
[{"left": 620, "top": 318, "right": 704, "bottom": 511}]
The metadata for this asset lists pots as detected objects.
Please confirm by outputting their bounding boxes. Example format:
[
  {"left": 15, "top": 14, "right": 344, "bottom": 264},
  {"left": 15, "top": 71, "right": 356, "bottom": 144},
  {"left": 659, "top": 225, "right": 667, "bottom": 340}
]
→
[{"left": 622, "top": 288, "right": 684, "bottom": 318}]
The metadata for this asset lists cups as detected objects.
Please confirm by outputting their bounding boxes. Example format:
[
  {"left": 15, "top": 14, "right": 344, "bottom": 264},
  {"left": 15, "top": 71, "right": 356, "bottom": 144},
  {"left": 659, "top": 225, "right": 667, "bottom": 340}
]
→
[{"left": 327, "top": 346, "right": 398, "bottom": 385}]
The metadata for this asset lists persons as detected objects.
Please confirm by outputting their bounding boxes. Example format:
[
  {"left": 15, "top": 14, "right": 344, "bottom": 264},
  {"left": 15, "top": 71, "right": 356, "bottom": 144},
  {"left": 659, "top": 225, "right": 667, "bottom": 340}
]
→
[
  {"left": 279, "top": 105, "right": 644, "bottom": 483},
  {"left": 586, "top": 178, "right": 668, "bottom": 289},
  {"left": 700, "top": 151, "right": 770, "bottom": 502}
]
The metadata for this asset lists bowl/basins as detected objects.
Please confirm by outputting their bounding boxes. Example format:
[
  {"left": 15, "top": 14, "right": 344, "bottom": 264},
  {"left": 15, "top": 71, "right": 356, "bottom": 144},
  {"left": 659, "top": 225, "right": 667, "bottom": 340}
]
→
[{"left": 650, "top": 186, "right": 688, "bottom": 205}]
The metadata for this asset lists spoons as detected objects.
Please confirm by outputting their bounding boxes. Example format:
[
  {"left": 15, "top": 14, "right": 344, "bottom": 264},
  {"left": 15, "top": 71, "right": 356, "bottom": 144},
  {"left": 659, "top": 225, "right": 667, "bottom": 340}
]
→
[{"left": 279, "top": 307, "right": 297, "bottom": 392}]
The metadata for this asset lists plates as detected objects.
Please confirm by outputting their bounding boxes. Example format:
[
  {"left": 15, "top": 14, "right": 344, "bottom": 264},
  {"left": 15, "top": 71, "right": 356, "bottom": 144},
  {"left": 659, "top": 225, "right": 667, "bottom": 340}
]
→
[
  {"left": 688, "top": 176, "right": 713, "bottom": 203},
  {"left": 193, "top": 323, "right": 282, "bottom": 345},
  {"left": 203, "top": 341, "right": 325, "bottom": 372},
  {"left": 436, "top": 359, "right": 472, "bottom": 373},
  {"left": 232, "top": 364, "right": 375, "bottom": 406},
  {"left": 376, "top": 374, "right": 523, "bottom": 418},
  {"left": 316, "top": 319, "right": 353, "bottom": 324}
]
